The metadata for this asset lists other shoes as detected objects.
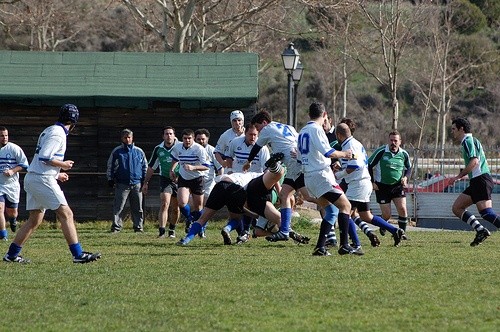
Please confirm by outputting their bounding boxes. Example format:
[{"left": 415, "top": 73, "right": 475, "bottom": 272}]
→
[
  {"left": 168, "top": 234, "right": 176, "bottom": 239},
  {"left": 156, "top": 234, "right": 165, "bottom": 239},
  {"left": 264, "top": 152, "right": 284, "bottom": 169}
]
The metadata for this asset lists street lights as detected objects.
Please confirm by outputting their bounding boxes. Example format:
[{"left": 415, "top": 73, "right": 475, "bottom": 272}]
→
[
  {"left": 291, "top": 59, "right": 304, "bottom": 132},
  {"left": 280, "top": 41, "right": 300, "bottom": 128}
]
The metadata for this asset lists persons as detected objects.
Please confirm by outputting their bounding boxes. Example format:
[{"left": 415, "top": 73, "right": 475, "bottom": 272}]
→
[
  {"left": 448, "top": 116, "right": 500, "bottom": 247},
  {"left": 186, "top": 128, "right": 225, "bottom": 224},
  {"left": 177, "top": 103, "right": 405, "bottom": 256},
  {"left": 0, "top": 125, "right": 30, "bottom": 239},
  {"left": 105, "top": 129, "right": 149, "bottom": 233},
  {"left": 424, "top": 168, "right": 432, "bottom": 181},
  {"left": 170, "top": 128, "right": 209, "bottom": 238},
  {"left": 3, "top": 103, "right": 101, "bottom": 265},
  {"left": 366, "top": 129, "right": 411, "bottom": 242},
  {"left": 142, "top": 126, "right": 187, "bottom": 239}
]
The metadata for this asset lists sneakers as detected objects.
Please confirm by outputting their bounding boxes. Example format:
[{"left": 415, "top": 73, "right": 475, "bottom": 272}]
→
[
  {"left": 338, "top": 244, "right": 360, "bottom": 255},
  {"left": 400, "top": 233, "right": 412, "bottom": 241},
  {"left": 369, "top": 233, "right": 381, "bottom": 247},
  {"left": 351, "top": 245, "right": 364, "bottom": 255},
  {"left": 220, "top": 228, "right": 232, "bottom": 245},
  {"left": 470, "top": 228, "right": 492, "bottom": 247},
  {"left": 265, "top": 230, "right": 289, "bottom": 242},
  {"left": 236, "top": 230, "right": 249, "bottom": 243},
  {"left": 170, "top": 240, "right": 188, "bottom": 247},
  {"left": 393, "top": 228, "right": 404, "bottom": 246},
  {"left": 185, "top": 216, "right": 194, "bottom": 233},
  {"left": 198, "top": 232, "right": 207, "bottom": 239},
  {"left": 312, "top": 247, "right": 332, "bottom": 256},
  {"left": 71, "top": 251, "right": 101, "bottom": 264},
  {"left": 8, "top": 217, "right": 17, "bottom": 232},
  {"left": 289, "top": 231, "right": 310, "bottom": 245},
  {"left": 1, "top": 236, "right": 9, "bottom": 242},
  {"left": 3, "top": 253, "right": 29, "bottom": 264},
  {"left": 379, "top": 227, "right": 386, "bottom": 235}
]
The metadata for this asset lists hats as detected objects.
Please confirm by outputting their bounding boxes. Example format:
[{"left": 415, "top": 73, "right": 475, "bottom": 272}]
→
[{"left": 230, "top": 110, "right": 245, "bottom": 122}]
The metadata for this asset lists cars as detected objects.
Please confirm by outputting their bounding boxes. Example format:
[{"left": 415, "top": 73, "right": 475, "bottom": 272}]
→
[{"left": 403, "top": 175, "right": 500, "bottom": 194}]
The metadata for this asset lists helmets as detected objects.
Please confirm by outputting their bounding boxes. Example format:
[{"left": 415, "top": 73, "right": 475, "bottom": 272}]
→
[{"left": 57, "top": 103, "right": 79, "bottom": 125}]
